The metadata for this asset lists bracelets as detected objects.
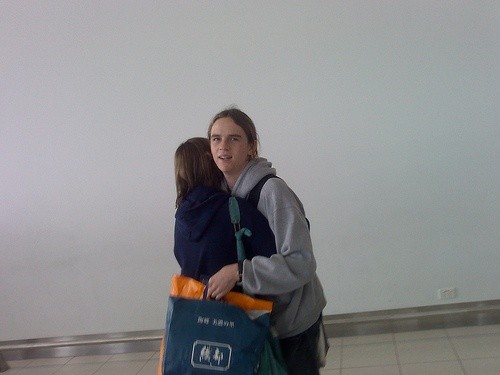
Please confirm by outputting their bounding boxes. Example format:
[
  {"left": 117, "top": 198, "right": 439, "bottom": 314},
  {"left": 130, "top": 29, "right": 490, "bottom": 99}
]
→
[{"left": 238, "top": 260, "right": 243, "bottom": 282}]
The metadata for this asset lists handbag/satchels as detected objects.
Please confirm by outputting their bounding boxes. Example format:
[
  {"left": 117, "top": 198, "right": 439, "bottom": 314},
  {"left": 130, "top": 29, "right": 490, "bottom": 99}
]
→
[
  {"left": 158, "top": 274, "right": 273, "bottom": 374},
  {"left": 162, "top": 296, "right": 269, "bottom": 375}
]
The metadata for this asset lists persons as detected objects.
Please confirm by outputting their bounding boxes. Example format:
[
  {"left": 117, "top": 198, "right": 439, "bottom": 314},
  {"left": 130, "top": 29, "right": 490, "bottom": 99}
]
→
[
  {"left": 173, "top": 138, "right": 289, "bottom": 375},
  {"left": 206, "top": 109, "right": 332, "bottom": 374}
]
